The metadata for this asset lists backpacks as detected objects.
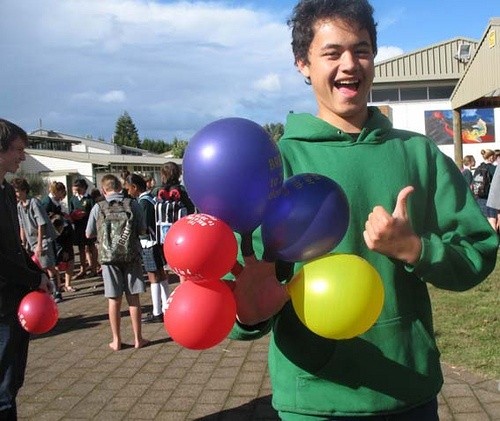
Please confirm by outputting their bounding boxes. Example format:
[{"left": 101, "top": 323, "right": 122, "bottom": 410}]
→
[
  {"left": 139, "top": 194, "right": 188, "bottom": 248},
  {"left": 92, "top": 197, "right": 144, "bottom": 266},
  {"left": 470, "top": 164, "right": 494, "bottom": 199}
]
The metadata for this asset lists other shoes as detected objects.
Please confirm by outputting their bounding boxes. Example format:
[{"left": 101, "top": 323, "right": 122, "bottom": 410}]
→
[
  {"left": 139, "top": 312, "right": 164, "bottom": 322},
  {"left": 86, "top": 271, "right": 97, "bottom": 278},
  {"left": 61, "top": 285, "right": 76, "bottom": 292},
  {"left": 73, "top": 271, "right": 85, "bottom": 279},
  {"left": 52, "top": 293, "right": 62, "bottom": 303}
]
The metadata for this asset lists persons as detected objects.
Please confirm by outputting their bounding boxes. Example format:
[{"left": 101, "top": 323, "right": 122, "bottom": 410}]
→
[
  {"left": 219, "top": 0, "right": 500, "bottom": 421},
  {"left": 0, "top": 118, "right": 197, "bottom": 421},
  {"left": 459, "top": 149, "right": 500, "bottom": 238}
]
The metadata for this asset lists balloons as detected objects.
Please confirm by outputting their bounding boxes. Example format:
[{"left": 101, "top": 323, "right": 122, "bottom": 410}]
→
[
  {"left": 162, "top": 117, "right": 385, "bottom": 350},
  {"left": 19, "top": 292, "right": 58, "bottom": 334}
]
